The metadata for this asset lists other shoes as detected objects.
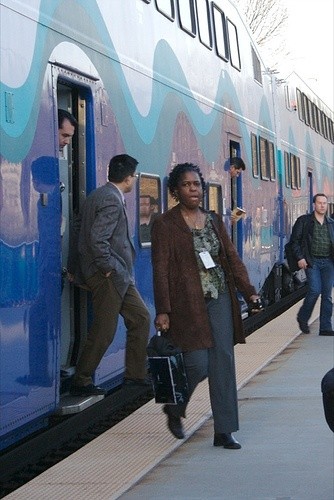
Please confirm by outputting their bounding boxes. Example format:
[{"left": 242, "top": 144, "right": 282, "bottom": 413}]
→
[
  {"left": 59, "top": 370, "right": 69, "bottom": 378},
  {"left": 296, "top": 317, "right": 310, "bottom": 334},
  {"left": 68, "top": 383, "right": 107, "bottom": 396},
  {"left": 319, "top": 330, "right": 334, "bottom": 337},
  {"left": 123, "top": 376, "right": 155, "bottom": 390}
]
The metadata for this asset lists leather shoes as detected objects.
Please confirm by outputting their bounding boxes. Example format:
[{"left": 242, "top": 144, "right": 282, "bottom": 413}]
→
[
  {"left": 214, "top": 432, "right": 242, "bottom": 450},
  {"left": 167, "top": 417, "right": 184, "bottom": 439}
]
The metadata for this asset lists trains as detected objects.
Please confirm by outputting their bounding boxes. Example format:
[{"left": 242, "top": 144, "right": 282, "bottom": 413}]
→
[{"left": 0, "top": 0, "right": 334, "bottom": 456}]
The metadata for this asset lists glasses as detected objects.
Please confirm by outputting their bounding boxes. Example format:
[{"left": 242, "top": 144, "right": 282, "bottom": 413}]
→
[{"left": 130, "top": 174, "right": 139, "bottom": 179}]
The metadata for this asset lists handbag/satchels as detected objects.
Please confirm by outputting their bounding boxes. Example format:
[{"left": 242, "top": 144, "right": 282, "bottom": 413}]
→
[
  {"left": 284, "top": 240, "right": 314, "bottom": 273},
  {"left": 147, "top": 330, "right": 191, "bottom": 406}
]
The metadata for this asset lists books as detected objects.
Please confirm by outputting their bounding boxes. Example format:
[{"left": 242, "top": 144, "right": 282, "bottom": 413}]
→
[{"left": 232, "top": 207, "right": 246, "bottom": 222}]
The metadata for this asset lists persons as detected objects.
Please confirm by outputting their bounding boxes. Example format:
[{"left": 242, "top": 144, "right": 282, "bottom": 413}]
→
[
  {"left": 139, "top": 196, "right": 159, "bottom": 243},
  {"left": 16, "top": 156, "right": 61, "bottom": 388},
  {"left": 154, "top": 163, "right": 261, "bottom": 449},
  {"left": 58, "top": 108, "right": 78, "bottom": 296},
  {"left": 290, "top": 194, "right": 334, "bottom": 336},
  {"left": 231, "top": 157, "right": 245, "bottom": 223},
  {"left": 68, "top": 155, "right": 154, "bottom": 396}
]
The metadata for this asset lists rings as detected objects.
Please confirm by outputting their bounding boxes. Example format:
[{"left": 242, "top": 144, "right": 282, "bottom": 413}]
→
[
  {"left": 158, "top": 327, "right": 161, "bottom": 328},
  {"left": 163, "top": 325, "right": 167, "bottom": 329}
]
